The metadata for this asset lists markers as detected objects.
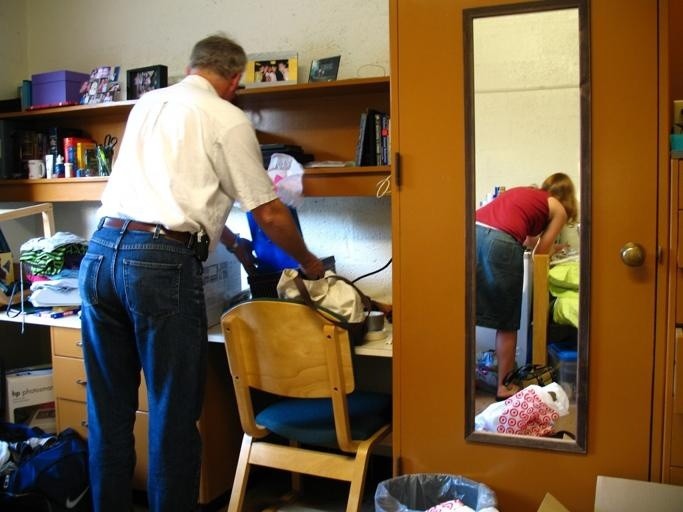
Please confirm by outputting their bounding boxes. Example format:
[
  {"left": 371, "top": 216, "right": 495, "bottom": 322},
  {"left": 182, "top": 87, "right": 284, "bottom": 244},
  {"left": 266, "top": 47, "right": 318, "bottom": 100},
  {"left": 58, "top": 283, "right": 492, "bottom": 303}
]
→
[{"left": 51, "top": 309, "right": 77, "bottom": 318}]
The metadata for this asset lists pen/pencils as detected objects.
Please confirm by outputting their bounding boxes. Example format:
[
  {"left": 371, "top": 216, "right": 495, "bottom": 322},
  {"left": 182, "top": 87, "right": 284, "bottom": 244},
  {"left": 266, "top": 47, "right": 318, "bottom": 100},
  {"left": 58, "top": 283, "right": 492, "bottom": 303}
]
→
[{"left": 97, "top": 145, "right": 110, "bottom": 177}]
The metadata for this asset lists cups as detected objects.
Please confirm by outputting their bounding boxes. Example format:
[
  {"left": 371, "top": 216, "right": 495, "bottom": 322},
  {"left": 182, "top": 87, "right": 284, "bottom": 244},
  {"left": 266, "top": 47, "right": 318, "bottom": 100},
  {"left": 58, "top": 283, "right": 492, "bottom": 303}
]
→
[
  {"left": 365, "top": 311, "right": 385, "bottom": 332},
  {"left": 98, "top": 156, "right": 111, "bottom": 176},
  {"left": 27, "top": 158, "right": 46, "bottom": 180}
]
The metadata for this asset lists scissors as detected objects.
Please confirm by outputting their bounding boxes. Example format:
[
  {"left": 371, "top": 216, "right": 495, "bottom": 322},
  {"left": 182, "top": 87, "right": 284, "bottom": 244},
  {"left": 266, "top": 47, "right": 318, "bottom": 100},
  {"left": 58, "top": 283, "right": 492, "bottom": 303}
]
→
[{"left": 104, "top": 134, "right": 118, "bottom": 149}]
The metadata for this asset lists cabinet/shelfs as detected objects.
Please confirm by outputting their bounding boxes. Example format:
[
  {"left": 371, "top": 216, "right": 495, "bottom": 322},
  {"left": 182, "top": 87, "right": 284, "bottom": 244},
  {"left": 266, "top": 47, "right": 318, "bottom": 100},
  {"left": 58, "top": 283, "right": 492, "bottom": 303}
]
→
[
  {"left": 1, "top": 74, "right": 393, "bottom": 505},
  {"left": 387, "top": 1, "right": 683, "bottom": 512}
]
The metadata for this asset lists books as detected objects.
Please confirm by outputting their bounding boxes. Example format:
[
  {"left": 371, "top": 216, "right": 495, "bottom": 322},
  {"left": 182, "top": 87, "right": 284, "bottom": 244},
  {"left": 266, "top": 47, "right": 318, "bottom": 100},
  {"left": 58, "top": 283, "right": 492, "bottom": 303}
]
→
[
  {"left": 76, "top": 141, "right": 98, "bottom": 169},
  {"left": 11, "top": 302, "right": 53, "bottom": 314},
  {"left": 50, "top": 121, "right": 70, "bottom": 173},
  {"left": 61, "top": 136, "right": 92, "bottom": 163},
  {"left": 376, "top": 113, "right": 382, "bottom": 166},
  {"left": 0, "top": 119, "right": 49, "bottom": 179},
  {"left": 382, "top": 117, "right": 390, "bottom": 164},
  {"left": 389, "top": 119, "right": 392, "bottom": 166},
  {"left": 356, "top": 107, "right": 381, "bottom": 167}
]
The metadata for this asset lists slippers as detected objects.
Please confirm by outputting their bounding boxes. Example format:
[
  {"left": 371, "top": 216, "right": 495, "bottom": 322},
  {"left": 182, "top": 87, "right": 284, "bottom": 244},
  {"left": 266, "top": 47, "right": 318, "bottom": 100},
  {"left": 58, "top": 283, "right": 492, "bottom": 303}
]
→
[{"left": 495, "top": 386, "right": 523, "bottom": 401}]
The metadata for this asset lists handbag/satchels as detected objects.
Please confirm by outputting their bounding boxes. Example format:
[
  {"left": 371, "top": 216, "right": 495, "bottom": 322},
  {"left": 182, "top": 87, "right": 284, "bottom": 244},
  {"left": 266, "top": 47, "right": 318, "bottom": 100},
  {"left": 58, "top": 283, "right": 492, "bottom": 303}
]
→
[
  {"left": 502, "top": 365, "right": 558, "bottom": 388},
  {"left": 279, "top": 267, "right": 369, "bottom": 345},
  {"left": 0, "top": 423, "right": 89, "bottom": 512}
]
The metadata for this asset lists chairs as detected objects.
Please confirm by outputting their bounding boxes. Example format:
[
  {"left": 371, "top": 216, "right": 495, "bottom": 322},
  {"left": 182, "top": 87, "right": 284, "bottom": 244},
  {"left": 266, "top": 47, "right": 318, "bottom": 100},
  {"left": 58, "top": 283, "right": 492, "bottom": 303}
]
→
[{"left": 217, "top": 299, "right": 391, "bottom": 511}]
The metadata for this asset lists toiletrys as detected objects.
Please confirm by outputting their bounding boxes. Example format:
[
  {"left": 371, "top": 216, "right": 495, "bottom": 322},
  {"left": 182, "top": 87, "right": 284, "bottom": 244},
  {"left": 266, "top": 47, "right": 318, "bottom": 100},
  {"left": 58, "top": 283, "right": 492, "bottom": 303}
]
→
[{"left": 55, "top": 153, "right": 65, "bottom": 178}]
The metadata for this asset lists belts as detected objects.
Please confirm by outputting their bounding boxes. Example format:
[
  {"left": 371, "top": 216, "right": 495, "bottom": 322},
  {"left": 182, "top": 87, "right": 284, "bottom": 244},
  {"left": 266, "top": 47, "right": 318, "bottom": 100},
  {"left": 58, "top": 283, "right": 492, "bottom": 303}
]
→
[{"left": 101, "top": 218, "right": 194, "bottom": 245}]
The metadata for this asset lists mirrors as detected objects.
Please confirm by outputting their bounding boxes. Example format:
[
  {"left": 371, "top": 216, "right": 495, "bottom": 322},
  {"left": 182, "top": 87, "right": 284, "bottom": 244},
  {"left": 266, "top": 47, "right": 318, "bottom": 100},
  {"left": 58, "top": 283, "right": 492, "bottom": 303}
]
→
[{"left": 462, "top": 1, "right": 591, "bottom": 455}]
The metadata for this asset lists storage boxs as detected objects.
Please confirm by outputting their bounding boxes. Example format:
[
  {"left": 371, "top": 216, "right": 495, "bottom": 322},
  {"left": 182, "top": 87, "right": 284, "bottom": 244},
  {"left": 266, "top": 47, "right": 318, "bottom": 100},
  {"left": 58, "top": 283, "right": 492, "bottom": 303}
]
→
[
  {"left": 3, "top": 365, "right": 57, "bottom": 435},
  {"left": 29, "top": 69, "right": 89, "bottom": 109}
]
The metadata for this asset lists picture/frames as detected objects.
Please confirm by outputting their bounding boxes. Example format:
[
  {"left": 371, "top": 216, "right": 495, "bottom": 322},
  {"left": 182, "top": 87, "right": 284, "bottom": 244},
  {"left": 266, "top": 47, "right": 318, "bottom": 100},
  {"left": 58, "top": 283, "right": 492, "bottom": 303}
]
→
[
  {"left": 125, "top": 65, "right": 167, "bottom": 100},
  {"left": 244, "top": 50, "right": 298, "bottom": 89},
  {"left": 307, "top": 56, "right": 341, "bottom": 82}
]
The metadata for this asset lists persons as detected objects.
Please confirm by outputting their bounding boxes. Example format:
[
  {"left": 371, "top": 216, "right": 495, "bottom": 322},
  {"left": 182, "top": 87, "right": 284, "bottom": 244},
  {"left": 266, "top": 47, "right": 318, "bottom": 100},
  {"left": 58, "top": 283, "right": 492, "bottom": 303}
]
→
[
  {"left": 80, "top": 67, "right": 119, "bottom": 105},
  {"left": 255, "top": 60, "right": 288, "bottom": 82},
  {"left": 476, "top": 173, "right": 579, "bottom": 400},
  {"left": 80, "top": 36, "right": 325, "bottom": 512}
]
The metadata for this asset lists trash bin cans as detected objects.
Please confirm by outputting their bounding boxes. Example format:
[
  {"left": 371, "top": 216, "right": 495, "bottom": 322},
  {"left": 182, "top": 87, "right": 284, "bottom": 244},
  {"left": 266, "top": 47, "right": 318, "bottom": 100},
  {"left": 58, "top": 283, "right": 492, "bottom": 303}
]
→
[{"left": 376, "top": 474, "right": 498, "bottom": 512}]
